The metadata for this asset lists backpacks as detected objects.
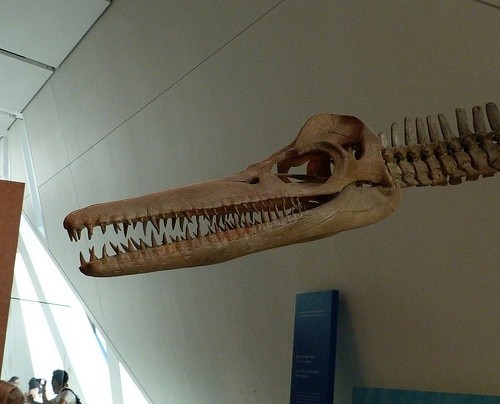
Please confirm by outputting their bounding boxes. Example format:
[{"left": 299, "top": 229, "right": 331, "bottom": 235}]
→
[{"left": 62, "top": 389, "right": 81, "bottom": 404}]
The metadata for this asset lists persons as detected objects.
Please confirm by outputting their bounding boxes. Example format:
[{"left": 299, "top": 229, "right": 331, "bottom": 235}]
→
[
  {"left": 27, "top": 370, "right": 82, "bottom": 404},
  {"left": 8, "top": 376, "right": 21, "bottom": 388}
]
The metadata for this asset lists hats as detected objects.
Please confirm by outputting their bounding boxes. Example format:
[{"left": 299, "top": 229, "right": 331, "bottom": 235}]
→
[{"left": 28, "top": 377, "right": 42, "bottom": 391}]
